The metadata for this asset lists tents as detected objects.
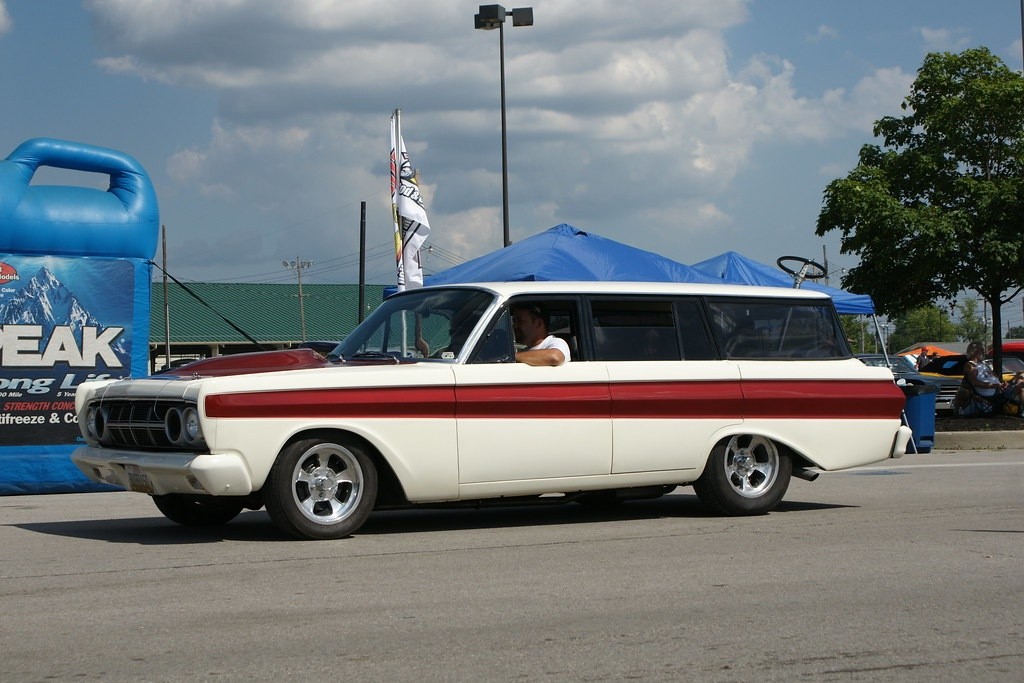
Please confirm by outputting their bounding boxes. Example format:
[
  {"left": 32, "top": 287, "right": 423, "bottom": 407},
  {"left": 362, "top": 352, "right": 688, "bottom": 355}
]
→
[{"left": 381, "top": 223, "right": 1024, "bottom": 454}]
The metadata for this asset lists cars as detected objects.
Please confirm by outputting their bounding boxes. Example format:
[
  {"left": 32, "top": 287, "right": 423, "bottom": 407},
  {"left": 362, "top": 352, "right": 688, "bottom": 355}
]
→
[
  {"left": 981, "top": 358, "right": 1024, "bottom": 416},
  {"left": 69, "top": 281, "right": 911, "bottom": 542}
]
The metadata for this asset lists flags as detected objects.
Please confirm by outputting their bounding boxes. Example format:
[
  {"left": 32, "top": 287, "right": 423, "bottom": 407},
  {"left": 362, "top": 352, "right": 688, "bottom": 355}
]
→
[{"left": 390, "top": 117, "right": 430, "bottom": 293}]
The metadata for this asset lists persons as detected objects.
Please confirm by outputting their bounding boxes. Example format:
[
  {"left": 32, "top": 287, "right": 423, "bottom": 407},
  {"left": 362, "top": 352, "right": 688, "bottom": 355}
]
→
[
  {"left": 964, "top": 342, "right": 1024, "bottom": 416},
  {"left": 490, "top": 304, "right": 571, "bottom": 366},
  {"left": 915, "top": 347, "right": 936, "bottom": 370}
]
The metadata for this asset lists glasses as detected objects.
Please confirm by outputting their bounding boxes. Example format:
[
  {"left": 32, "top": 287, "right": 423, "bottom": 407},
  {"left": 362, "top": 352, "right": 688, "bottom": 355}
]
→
[{"left": 448, "top": 327, "right": 466, "bottom": 337}]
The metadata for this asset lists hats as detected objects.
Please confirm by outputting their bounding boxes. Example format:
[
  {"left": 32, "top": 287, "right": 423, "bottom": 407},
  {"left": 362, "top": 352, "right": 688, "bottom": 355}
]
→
[{"left": 922, "top": 347, "right": 929, "bottom": 351}]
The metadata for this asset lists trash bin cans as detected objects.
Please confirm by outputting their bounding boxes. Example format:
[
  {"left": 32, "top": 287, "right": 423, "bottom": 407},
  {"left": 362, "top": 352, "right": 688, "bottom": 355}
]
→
[{"left": 894, "top": 374, "right": 941, "bottom": 454}]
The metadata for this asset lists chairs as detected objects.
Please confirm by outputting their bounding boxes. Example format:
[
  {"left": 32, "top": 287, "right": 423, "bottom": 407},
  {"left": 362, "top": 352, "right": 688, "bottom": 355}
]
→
[
  {"left": 553, "top": 333, "right": 578, "bottom": 361},
  {"left": 963, "top": 376, "right": 1022, "bottom": 418}
]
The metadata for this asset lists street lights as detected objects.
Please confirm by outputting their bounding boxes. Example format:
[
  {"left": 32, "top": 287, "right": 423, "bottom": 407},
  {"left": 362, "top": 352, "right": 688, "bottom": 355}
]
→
[
  {"left": 282, "top": 255, "right": 315, "bottom": 342},
  {"left": 473, "top": 3, "right": 535, "bottom": 248}
]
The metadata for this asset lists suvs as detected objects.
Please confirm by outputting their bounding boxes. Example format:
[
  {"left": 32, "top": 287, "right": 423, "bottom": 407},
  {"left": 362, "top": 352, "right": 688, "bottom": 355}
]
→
[{"left": 855, "top": 353, "right": 963, "bottom": 403}]
